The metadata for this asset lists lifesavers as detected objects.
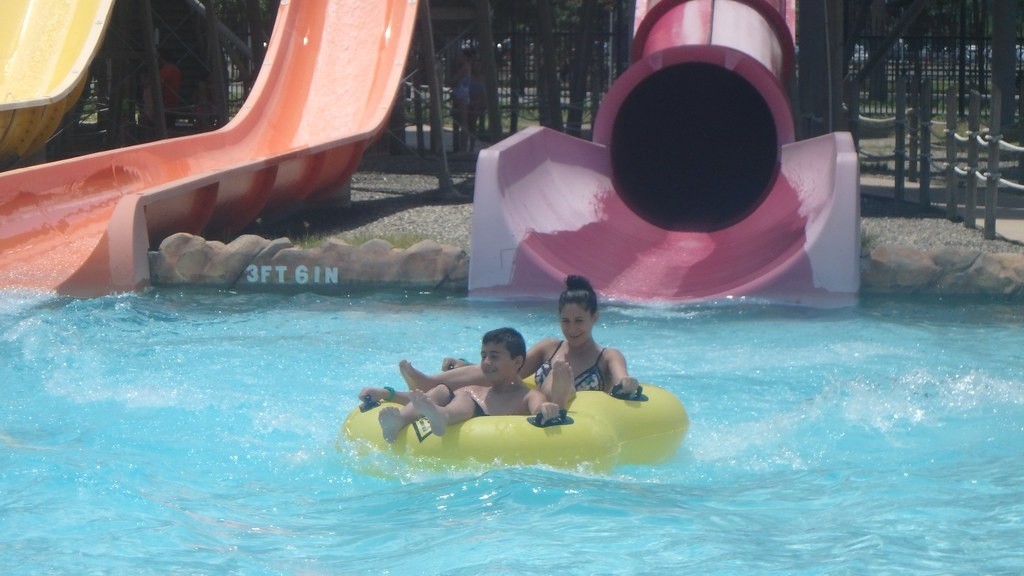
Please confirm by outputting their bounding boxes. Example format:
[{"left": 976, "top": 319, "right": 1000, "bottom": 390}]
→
[{"left": 341, "top": 373, "right": 689, "bottom": 477}]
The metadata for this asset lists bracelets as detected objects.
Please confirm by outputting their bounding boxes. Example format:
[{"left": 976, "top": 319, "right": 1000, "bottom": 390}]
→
[
  {"left": 458, "top": 358, "right": 470, "bottom": 366},
  {"left": 383, "top": 386, "right": 395, "bottom": 401}
]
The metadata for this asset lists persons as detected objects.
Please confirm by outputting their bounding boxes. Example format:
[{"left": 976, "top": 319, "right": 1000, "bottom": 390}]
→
[
  {"left": 441, "top": 276, "right": 638, "bottom": 395},
  {"left": 446, "top": 49, "right": 470, "bottom": 152},
  {"left": 141, "top": 47, "right": 182, "bottom": 128},
  {"left": 464, "top": 47, "right": 484, "bottom": 145},
  {"left": 358, "top": 327, "right": 561, "bottom": 443}
]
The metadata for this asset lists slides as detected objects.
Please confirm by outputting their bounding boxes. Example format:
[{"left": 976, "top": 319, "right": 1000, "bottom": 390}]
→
[
  {"left": 1, "top": 1, "right": 421, "bottom": 295},
  {"left": 468, "top": 1, "right": 860, "bottom": 310}
]
